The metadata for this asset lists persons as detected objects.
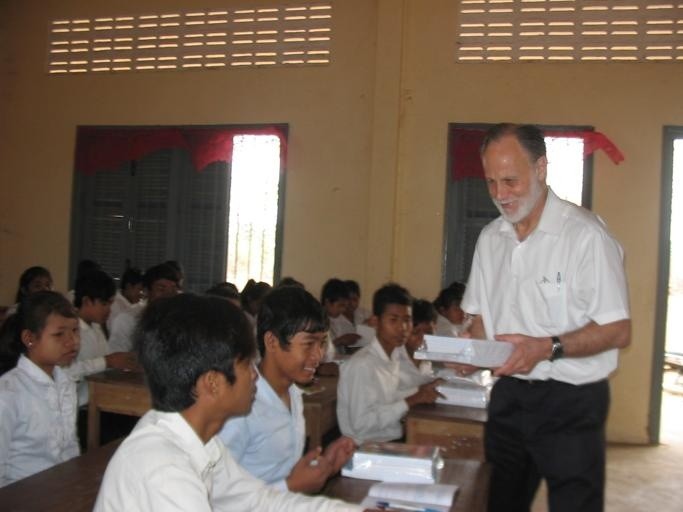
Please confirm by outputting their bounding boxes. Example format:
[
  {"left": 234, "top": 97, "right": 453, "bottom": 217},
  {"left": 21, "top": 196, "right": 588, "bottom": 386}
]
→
[
  {"left": 450, "top": 122, "right": 633, "bottom": 512},
  {"left": 3, "top": 261, "right": 184, "bottom": 381},
  {"left": 0, "top": 290, "right": 82, "bottom": 487},
  {"left": 206, "top": 277, "right": 477, "bottom": 384},
  {"left": 336, "top": 280, "right": 448, "bottom": 446},
  {"left": 91, "top": 293, "right": 406, "bottom": 512},
  {"left": 213, "top": 283, "right": 358, "bottom": 495}
]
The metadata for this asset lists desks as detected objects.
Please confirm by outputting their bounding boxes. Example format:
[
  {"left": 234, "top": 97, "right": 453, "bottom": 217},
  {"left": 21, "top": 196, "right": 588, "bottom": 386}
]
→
[
  {"left": 2, "top": 437, "right": 125, "bottom": 512},
  {"left": 88, "top": 358, "right": 485, "bottom": 452},
  {"left": 323, "top": 433, "right": 486, "bottom": 510}
]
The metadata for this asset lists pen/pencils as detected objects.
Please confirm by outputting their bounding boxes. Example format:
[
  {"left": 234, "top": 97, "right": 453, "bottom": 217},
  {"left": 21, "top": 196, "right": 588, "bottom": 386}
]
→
[
  {"left": 378, "top": 502, "right": 444, "bottom": 512},
  {"left": 434, "top": 388, "right": 446, "bottom": 400}
]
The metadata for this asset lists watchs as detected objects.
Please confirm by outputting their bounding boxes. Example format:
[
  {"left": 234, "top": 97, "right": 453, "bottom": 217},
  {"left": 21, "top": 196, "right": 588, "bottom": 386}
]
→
[{"left": 550, "top": 335, "right": 564, "bottom": 363}]
